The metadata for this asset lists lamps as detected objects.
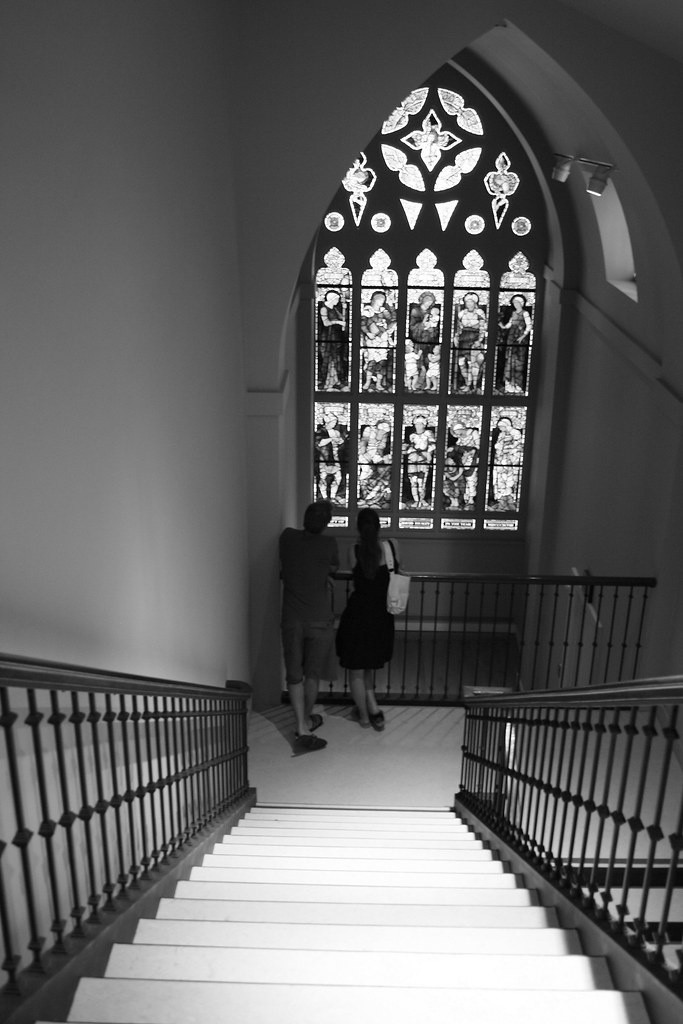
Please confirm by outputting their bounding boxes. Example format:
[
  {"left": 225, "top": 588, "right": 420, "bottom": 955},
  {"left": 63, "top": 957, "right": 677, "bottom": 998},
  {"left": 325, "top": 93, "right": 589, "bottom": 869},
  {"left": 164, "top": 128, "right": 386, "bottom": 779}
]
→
[
  {"left": 551, "top": 157, "right": 572, "bottom": 183},
  {"left": 586, "top": 166, "right": 610, "bottom": 196}
]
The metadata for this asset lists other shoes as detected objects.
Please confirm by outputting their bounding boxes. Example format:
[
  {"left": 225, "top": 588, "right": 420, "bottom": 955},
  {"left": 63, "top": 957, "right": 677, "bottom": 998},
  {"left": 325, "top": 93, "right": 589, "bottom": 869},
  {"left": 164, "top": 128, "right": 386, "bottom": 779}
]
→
[
  {"left": 293, "top": 714, "right": 323, "bottom": 737},
  {"left": 292, "top": 735, "right": 328, "bottom": 754},
  {"left": 351, "top": 706, "right": 370, "bottom": 728},
  {"left": 369, "top": 709, "right": 384, "bottom": 730}
]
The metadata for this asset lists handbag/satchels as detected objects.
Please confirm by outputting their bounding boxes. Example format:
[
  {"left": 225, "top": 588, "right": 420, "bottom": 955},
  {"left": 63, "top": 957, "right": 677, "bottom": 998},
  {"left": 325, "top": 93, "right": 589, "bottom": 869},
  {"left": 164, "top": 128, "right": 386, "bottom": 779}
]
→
[{"left": 383, "top": 540, "right": 410, "bottom": 615}]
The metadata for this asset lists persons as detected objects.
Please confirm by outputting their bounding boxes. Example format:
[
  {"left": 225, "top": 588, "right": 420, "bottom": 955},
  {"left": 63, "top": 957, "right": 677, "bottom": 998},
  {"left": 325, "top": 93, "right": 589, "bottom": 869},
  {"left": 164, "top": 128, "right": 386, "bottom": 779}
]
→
[
  {"left": 313, "top": 403, "right": 525, "bottom": 513},
  {"left": 315, "top": 289, "right": 533, "bottom": 397},
  {"left": 273, "top": 500, "right": 341, "bottom": 758},
  {"left": 334, "top": 508, "right": 411, "bottom": 732}
]
